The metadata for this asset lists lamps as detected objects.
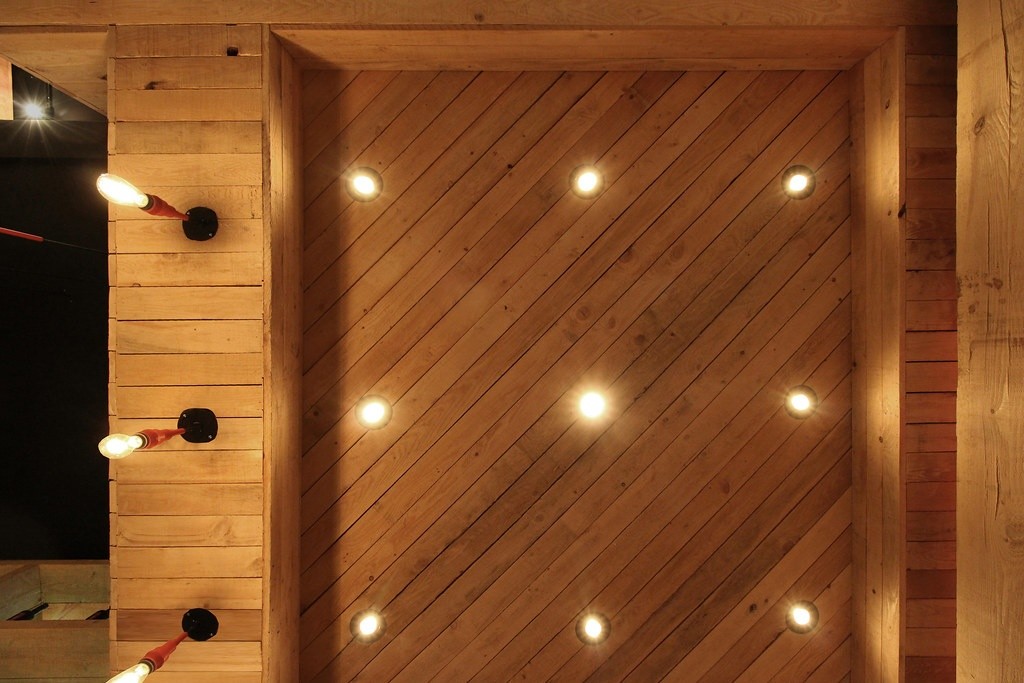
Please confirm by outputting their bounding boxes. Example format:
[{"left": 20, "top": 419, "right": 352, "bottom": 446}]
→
[
  {"left": 104, "top": 608, "right": 222, "bottom": 683},
  {"left": 97, "top": 407, "right": 220, "bottom": 459},
  {"left": 97, "top": 171, "right": 219, "bottom": 242}
]
